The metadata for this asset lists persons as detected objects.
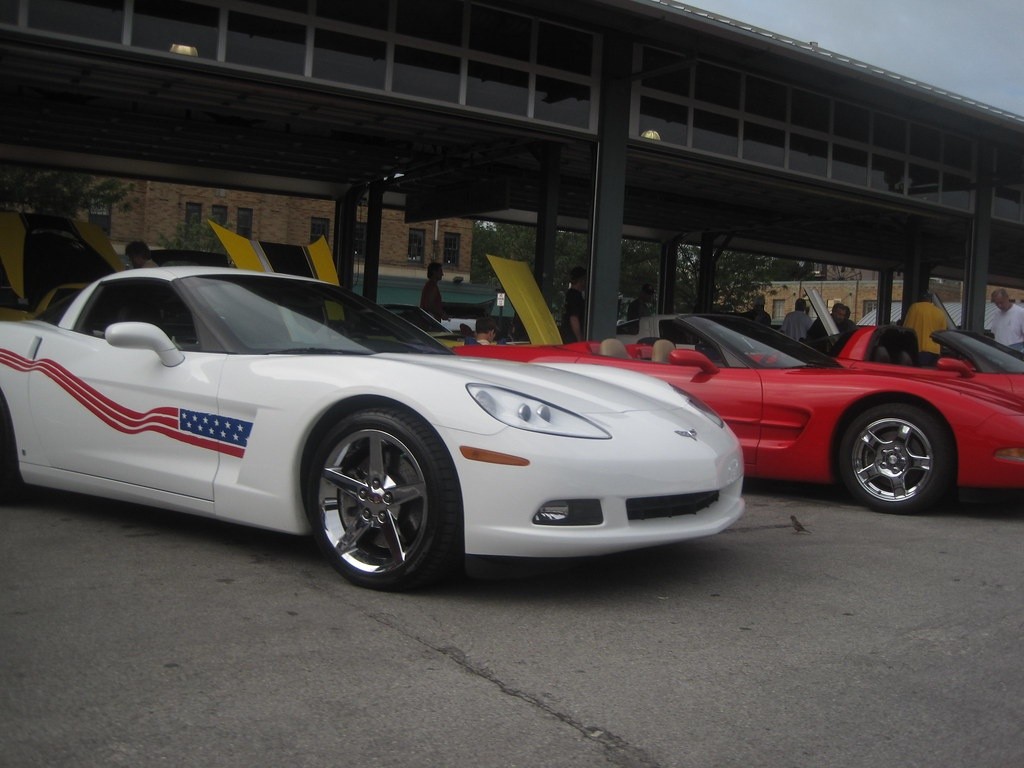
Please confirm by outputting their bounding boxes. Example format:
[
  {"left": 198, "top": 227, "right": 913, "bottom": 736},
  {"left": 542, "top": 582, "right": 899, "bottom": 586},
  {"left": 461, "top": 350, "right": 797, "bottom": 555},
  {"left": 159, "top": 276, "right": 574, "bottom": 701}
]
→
[
  {"left": 627, "top": 284, "right": 653, "bottom": 320},
  {"left": 465, "top": 316, "right": 506, "bottom": 346},
  {"left": 901, "top": 289, "right": 949, "bottom": 356},
  {"left": 832, "top": 302, "right": 846, "bottom": 323},
  {"left": 562, "top": 265, "right": 586, "bottom": 344},
  {"left": 990, "top": 288, "right": 1024, "bottom": 346},
  {"left": 126, "top": 240, "right": 158, "bottom": 269},
  {"left": 780, "top": 298, "right": 812, "bottom": 341},
  {"left": 420, "top": 262, "right": 449, "bottom": 322}
]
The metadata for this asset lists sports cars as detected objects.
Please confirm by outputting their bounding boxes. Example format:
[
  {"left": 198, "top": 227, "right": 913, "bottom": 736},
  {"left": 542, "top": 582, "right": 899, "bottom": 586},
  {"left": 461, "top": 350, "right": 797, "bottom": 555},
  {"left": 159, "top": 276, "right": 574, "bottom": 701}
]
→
[
  {"left": 447, "top": 310, "right": 1024, "bottom": 516},
  {"left": 0, "top": 266, "right": 745, "bottom": 593},
  {"left": 754, "top": 325, "right": 1024, "bottom": 399}
]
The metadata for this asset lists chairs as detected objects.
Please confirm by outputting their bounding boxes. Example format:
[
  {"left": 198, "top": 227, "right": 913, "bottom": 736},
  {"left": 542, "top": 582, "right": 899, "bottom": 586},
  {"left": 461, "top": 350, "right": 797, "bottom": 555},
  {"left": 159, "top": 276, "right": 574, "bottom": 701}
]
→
[
  {"left": 871, "top": 346, "right": 891, "bottom": 363},
  {"left": 161, "top": 292, "right": 197, "bottom": 345},
  {"left": 652, "top": 338, "right": 676, "bottom": 362},
  {"left": 599, "top": 338, "right": 633, "bottom": 360},
  {"left": 895, "top": 352, "right": 914, "bottom": 367}
]
